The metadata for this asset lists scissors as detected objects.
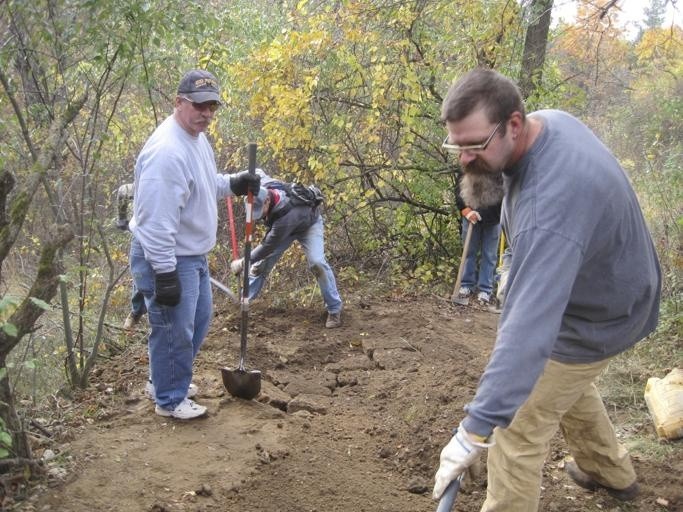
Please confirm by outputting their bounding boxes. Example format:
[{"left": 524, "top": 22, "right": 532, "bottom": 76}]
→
[{"left": 220, "top": 142, "right": 263, "bottom": 399}]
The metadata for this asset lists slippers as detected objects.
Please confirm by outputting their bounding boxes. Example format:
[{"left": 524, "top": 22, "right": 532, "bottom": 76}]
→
[{"left": 260, "top": 181, "right": 324, "bottom": 245}]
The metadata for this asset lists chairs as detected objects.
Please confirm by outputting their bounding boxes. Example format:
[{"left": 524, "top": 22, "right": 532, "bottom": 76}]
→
[
  {"left": 325, "top": 305, "right": 342, "bottom": 329},
  {"left": 154, "top": 397, "right": 208, "bottom": 419},
  {"left": 477, "top": 291, "right": 493, "bottom": 303},
  {"left": 458, "top": 286, "right": 471, "bottom": 298},
  {"left": 143, "top": 379, "right": 199, "bottom": 400},
  {"left": 123, "top": 311, "right": 141, "bottom": 331},
  {"left": 565, "top": 459, "right": 641, "bottom": 501}
]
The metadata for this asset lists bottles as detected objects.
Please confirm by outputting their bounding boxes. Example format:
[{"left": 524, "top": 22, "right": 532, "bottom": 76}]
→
[
  {"left": 243, "top": 185, "right": 268, "bottom": 221},
  {"left": 177, "top": 69, "right": 222, "bottom": 106}
]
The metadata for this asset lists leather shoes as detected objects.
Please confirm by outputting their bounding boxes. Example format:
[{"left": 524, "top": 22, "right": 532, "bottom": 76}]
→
[
  {"left": 230, "top": 169, "right": 260, "bottom": 197},
  {"left": 231, "top": 257, "right": 244, "bottom": 276},
  {"left": 461, "top": 207, "right": 482, "bottom": 225},
  {"left": 153, "top": 267, "right": 182, "bottom": 307},
  {"left": 431, "top": 420, "right": 496, "bottom": 502}
]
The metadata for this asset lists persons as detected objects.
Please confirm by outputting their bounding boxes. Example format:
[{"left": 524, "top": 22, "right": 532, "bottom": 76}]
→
[
  {"left": 231, "top": 168, "right": 341, "bottom": 327},
  {"left": 128, "top": 71, "right": 260, "bottom": 419},
  {"left": 114, "top": 183, "right": 146, "bottom": 329},
  {"left": 432, "top": 68, "right": 661, "bottom": 512},
  {"left": 454, "top": 168, "right": 500, "bottom": 302}
]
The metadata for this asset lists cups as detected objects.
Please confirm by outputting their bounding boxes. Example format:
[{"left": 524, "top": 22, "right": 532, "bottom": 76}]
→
[
  {"left": 191, "top": 102, "right": 220, "bottom": 112},
  {"left": 441, "top": 120, "right": 503, "bottom": 155}
]
